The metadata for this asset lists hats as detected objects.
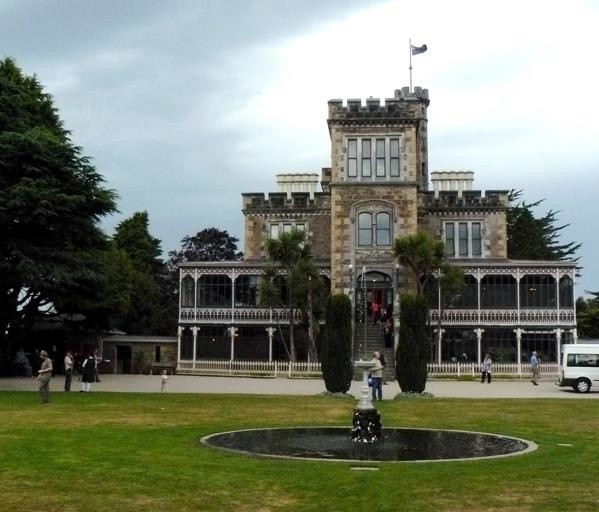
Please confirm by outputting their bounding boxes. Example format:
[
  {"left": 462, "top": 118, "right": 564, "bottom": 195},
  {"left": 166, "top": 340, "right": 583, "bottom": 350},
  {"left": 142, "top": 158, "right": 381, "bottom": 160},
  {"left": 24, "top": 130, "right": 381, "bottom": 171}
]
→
[{"left": 39, "top": 350, "right": 49, "bottom": 357}]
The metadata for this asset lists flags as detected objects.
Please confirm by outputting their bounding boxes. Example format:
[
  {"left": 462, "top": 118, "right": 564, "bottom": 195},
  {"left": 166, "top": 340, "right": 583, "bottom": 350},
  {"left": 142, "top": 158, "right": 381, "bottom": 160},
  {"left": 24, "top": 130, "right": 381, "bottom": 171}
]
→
[{"left": 411, "top": 44, "right": 427, "bottom": 55}]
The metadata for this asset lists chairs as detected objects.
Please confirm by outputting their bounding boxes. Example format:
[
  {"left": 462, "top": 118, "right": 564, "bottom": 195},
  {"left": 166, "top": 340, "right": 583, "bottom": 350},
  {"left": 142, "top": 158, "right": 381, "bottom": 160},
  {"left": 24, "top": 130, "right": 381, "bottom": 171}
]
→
[{"left": 595, "top": 359, "right": 599, "bottom": 366}]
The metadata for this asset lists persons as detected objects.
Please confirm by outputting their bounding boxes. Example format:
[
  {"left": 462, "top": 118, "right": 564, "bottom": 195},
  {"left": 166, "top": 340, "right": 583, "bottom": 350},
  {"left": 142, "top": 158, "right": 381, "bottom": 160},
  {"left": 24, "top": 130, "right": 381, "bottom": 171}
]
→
[
  {"left": 369, "top": 352, "right": 383, "bottom": 401},
  {"left": 81, "top": 350, "right": 94, "bottom": 392},
  {"left": 379, "top": 352, "right": 388, "bottom": 385},
  {"left": 482, "top": 354, "right": 492, "bottom": 383},
  {"left": 161, "top": 369, "right": 168, "bottom": 392},
  {"left": 64, "top": 351, "right": 74, "bottom": 391},
  {"left": 357, "top": 300, "right": 392, "bottom": 348},
  {"left": 94, "top": 347, "right": 104, "bottom": 382},
  {"left": 15, "top": 347, "right": 30, "bottom": 365},
  {"left": 530, "top": 351, "right": 540, "bottom": 385},
  {"left": 36, "top": 350, "right": 53, "bottom": 402}
]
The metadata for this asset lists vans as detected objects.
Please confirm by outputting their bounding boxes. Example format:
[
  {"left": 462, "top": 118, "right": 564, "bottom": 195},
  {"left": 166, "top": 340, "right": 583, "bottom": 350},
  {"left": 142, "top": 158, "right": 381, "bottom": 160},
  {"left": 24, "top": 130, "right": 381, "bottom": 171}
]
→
[{"left": 558, "top": 344, "right": 599, "bottom": 393}]
